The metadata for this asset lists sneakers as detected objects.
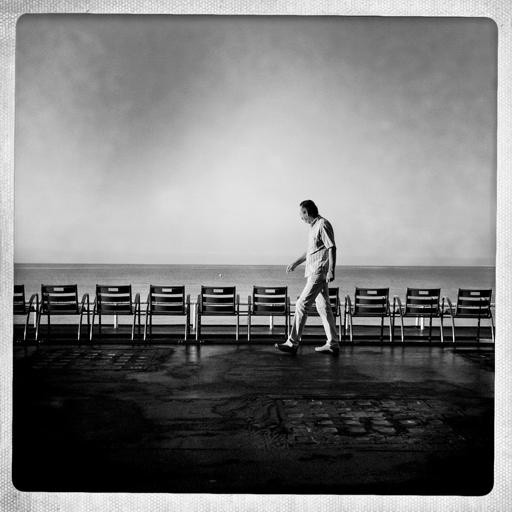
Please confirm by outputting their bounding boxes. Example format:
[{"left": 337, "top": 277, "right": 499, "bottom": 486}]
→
[
  {"left": 275, "top": 343, "right": 297, "bottom": 353},
  {"left": 314, "top": 343, "right": 338, "bottom": 354}
]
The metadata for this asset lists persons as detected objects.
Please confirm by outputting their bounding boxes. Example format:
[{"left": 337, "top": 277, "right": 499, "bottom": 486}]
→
[{"left": 274, "top": 200, "right": 340, "bottom": 355}]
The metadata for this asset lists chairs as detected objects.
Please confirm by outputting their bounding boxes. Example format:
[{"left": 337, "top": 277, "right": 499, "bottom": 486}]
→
[
  {"left": 88, "top": 283, "right": 141, "bottom": 341},
  {"left": 13, "top": 284, "right": 40, "bottom": 341},
  {"left": 247, "top": 286, "right": 292, "bottom": 341},
  {"left": 35, "top": 284, "right": 90, "bottom": 341},
  {"left": 392, "top": 288, "right": 443, "bottom": 343},
  {"left": 440, "top": 287, "right": 494, "bottom": 342},
  {"left": 298, "top": 288, "right": 341, "bottom": 341},
  {"left": 194, "top": 285, "right": 240, "bottom": 341},
  {"left": 344, "top": 287, "right": 393, "bottom": 343},
  {"left": 143, "top": 284, "right": 190, "bottom": 340}
]
